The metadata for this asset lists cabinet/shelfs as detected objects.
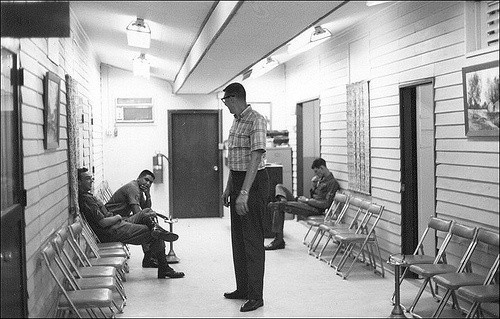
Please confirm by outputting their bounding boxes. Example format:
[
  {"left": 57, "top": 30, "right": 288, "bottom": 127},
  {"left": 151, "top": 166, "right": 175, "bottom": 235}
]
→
[{"left": 267, "top": 148, "right": 293, "bottom": 195}]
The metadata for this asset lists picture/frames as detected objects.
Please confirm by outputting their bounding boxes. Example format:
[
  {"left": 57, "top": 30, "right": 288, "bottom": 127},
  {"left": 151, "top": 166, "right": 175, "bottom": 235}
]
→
[
  {"left": 44, "top": 72, "right": 60, "bottom": 150},
  {"left": 462, "top": 60, "right": 500, "bottom": 138}
]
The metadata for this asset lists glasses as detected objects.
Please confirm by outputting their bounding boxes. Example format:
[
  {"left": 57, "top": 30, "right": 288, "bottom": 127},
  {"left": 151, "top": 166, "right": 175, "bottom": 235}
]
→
[{"left": 221, "top": 95, "right": 235, "bottom": 103}]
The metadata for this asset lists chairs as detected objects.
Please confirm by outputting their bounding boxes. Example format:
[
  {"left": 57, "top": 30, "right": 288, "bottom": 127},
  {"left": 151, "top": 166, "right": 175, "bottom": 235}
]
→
[
  {"left": 389, "top": 215, "right": 500, "bottom": 319},
  {"left": 40, "top": 180, "right": 131, "bottom": 319},
  {"left": 303, "top": 190, "right": 385, "bottom": 279}
]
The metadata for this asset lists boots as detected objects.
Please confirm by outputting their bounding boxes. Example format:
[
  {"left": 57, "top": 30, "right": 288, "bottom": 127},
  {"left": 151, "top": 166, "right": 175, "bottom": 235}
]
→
[
  {"left": 156, "top": 248, "right": 185, "bottom": 278},
  {"left": 143, "top": 215, "right": 179, "bottom": 242},
  {"left": 142, "top": 251, "right": 158, "bottom": 268}
]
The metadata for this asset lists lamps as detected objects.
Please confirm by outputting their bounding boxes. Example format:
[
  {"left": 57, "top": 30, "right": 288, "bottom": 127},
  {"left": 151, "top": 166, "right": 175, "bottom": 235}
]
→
[
  {"left": 287, "top": 26, "right": 333, "bottom": 54},
  {"left": 126, "top": 18, "right": 151, "bottom": 48}
]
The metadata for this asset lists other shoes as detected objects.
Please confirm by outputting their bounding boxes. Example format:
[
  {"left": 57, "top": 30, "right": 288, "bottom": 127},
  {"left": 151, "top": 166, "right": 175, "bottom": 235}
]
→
[{"left": 264, "top": 239, "right": 285, "bottom": 250}]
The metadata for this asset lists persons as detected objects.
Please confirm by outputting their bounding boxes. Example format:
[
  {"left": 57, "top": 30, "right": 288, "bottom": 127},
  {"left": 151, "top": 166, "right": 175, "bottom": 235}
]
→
[
  {"left": 221, "top": 84, "right": 267, "bottom": 312},
  {"left": 105, "top": 170, "right": 158, "bottom": 267},
  {"left": 78, "top": 167, "right": 184, "bottom": 279},
  {"left": 264, "top": 158, "right": 340, "bottom": 250}
]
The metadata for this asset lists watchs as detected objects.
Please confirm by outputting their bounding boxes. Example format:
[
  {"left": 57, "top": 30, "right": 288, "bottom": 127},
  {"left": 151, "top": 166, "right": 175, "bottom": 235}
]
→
[{"left": 240, "top": 190, "right": 248, "bottom": 196}]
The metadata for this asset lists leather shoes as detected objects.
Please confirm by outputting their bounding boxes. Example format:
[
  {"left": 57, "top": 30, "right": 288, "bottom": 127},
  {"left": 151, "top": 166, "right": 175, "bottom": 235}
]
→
[
  {"left": 224, "top": 290, "right": 247, "bottom": 299},
  {"left": 240, "top": 294, "right": 263, "bottom": 312}
]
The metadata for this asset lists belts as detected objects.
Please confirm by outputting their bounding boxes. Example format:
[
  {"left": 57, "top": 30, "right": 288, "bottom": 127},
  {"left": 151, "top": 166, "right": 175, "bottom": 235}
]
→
[{"left": 229, "top": 168, "right": 268, "bottom": 175}]
